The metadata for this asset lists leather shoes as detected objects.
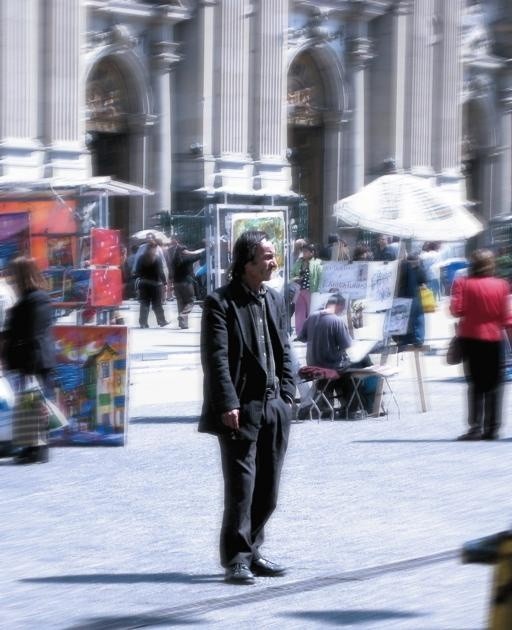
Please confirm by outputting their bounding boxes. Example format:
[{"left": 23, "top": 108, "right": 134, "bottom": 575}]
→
[
  {"left": 225, "top": 564, "right": 253, "bottom": 584},
  {"left": 252, "top": 557, "right": 285, "bottom": 575}
]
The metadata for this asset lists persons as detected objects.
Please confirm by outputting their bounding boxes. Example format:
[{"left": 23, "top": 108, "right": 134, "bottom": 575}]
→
[
  {"left": 449, "top": 249, "right": 511, "bottom": 440},
  {"left": 56, "top": 233, "right": 230, "bottom": 329},
  {"left": 266, "top": 232, "right": 443, "bottom": 351},
  {"left": 0, "top": 278, "right": 24, "bottom": 457},
  {"left": 300, "top": 294, "right": 370, "bottom": 417},
  {"left": 196, "top": 230, "right": 299, "bottom": 584},
  {"left": 0, "top": 256, "right": 57, "bottom": 466}
]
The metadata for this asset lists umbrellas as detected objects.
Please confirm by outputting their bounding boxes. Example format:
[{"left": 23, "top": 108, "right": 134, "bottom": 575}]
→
[{"left": 334, "top": 173, "right": 486, "bottom": 242}]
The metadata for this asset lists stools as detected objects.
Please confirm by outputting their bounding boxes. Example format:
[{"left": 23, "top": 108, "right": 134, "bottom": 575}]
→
[{"left": 292, "top": 363, "right": 400, "bottom": 422}]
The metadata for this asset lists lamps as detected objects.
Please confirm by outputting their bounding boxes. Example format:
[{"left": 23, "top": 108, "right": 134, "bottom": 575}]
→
[{"left": 190, "top": 142, "right": 204, "bottom": 155}]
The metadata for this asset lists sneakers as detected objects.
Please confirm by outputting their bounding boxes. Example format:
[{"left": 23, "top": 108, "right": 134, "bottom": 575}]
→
[
  {"left": 13, "top": 451, "right": 48, "bottom": 464},
  {"left": 141, "top": 316, "right": 189, "bottom": 329},
  {"left": 458, "top": 433, "right": 499, "bottom": 441}
]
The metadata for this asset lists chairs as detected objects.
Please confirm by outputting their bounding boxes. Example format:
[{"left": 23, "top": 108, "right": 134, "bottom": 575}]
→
[{"left": 39, "top": 265, "right": 91, "bottom": 317}]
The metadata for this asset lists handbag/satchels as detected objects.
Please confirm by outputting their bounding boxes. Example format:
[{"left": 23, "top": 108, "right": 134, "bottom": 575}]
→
[
  {"left": 448, "top": 338, "right": 462, "bottom": 365},
  {"left": 11, "top": 389, "right": 69, "bottom": 447},
  {"left": 420, "top": 287, "right": 436, "bottom": 313}
]
